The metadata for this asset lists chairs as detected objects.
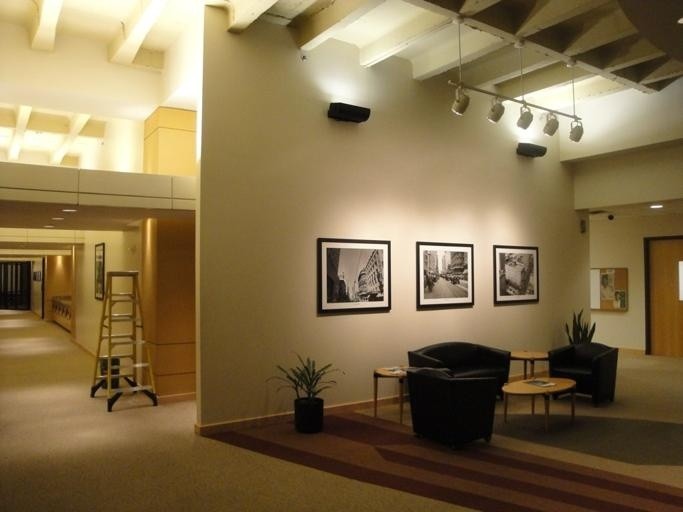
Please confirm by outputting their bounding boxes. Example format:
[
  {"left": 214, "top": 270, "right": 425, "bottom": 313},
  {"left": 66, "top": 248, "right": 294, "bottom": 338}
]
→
[
  {"left": 547, "top": 342, "right": 618, "bottom": 407},
  {"left": 408, "top": 341, "right": 511, "bottom": 402},
  {"left": 405, "top": 366, "right": 497, "bottom": 451}
]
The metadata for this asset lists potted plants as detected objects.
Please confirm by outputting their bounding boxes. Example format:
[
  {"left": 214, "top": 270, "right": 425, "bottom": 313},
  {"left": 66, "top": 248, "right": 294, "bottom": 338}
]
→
[{"left": 265, "top": 350, "right": 346, "bottom": 433}]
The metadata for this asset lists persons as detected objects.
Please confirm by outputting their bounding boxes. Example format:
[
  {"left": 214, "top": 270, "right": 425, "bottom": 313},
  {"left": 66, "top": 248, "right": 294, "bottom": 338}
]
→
[
  {"left": 528, "top": 379, "right": 556, "bottom": 386},
  {"left": 614, "top": 292, "right": 622, "bottom": 308},
  {"left": 600, "top": 275, "right": 613, "bottom": 299}
]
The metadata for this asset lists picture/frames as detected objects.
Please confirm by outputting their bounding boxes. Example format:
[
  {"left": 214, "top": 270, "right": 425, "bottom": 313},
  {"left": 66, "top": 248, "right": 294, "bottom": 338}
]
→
[
  {"left": 493, "top": 244, "right": 539, "bottom": 305},
  {"left": 416, "top": 241, "right": 474, "bottom": 311},
  {"left": 94, "top": 242, "right": 105, "bottom": 300},
  {"left": 316, "top": 237, "right": 392, "bottom": 314}
]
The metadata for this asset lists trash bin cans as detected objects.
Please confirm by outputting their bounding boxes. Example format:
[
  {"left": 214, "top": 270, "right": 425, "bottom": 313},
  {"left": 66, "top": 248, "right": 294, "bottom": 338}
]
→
[{"left": 100, "top": 358, "right": 119, "bottom": 389}]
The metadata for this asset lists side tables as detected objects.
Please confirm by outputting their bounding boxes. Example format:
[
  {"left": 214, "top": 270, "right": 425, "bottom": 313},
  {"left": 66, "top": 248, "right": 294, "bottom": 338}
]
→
[
  {"left": 373, "top": 366, "right": 408, "bottom": 425},
  {"left": 509, "top": 351, "right": 549, "bottom": 381}
]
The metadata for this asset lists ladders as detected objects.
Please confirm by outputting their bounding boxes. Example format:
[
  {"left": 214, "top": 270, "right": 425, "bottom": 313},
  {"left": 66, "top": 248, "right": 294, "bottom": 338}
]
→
[{"left": 91, "top": 272, "right": 159, "bottom": 412}]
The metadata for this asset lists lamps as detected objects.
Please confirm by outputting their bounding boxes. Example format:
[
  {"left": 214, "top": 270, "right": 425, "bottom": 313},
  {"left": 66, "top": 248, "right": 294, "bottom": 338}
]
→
[
  {"left": 515, "top": 143, "right": 547, "bottom": 158},
  {"left": 326, "top": 101, "right": 371, "bottom": 123},
  {"left": 447, "top": 16, "right": 583, "bottom": 142}
]
